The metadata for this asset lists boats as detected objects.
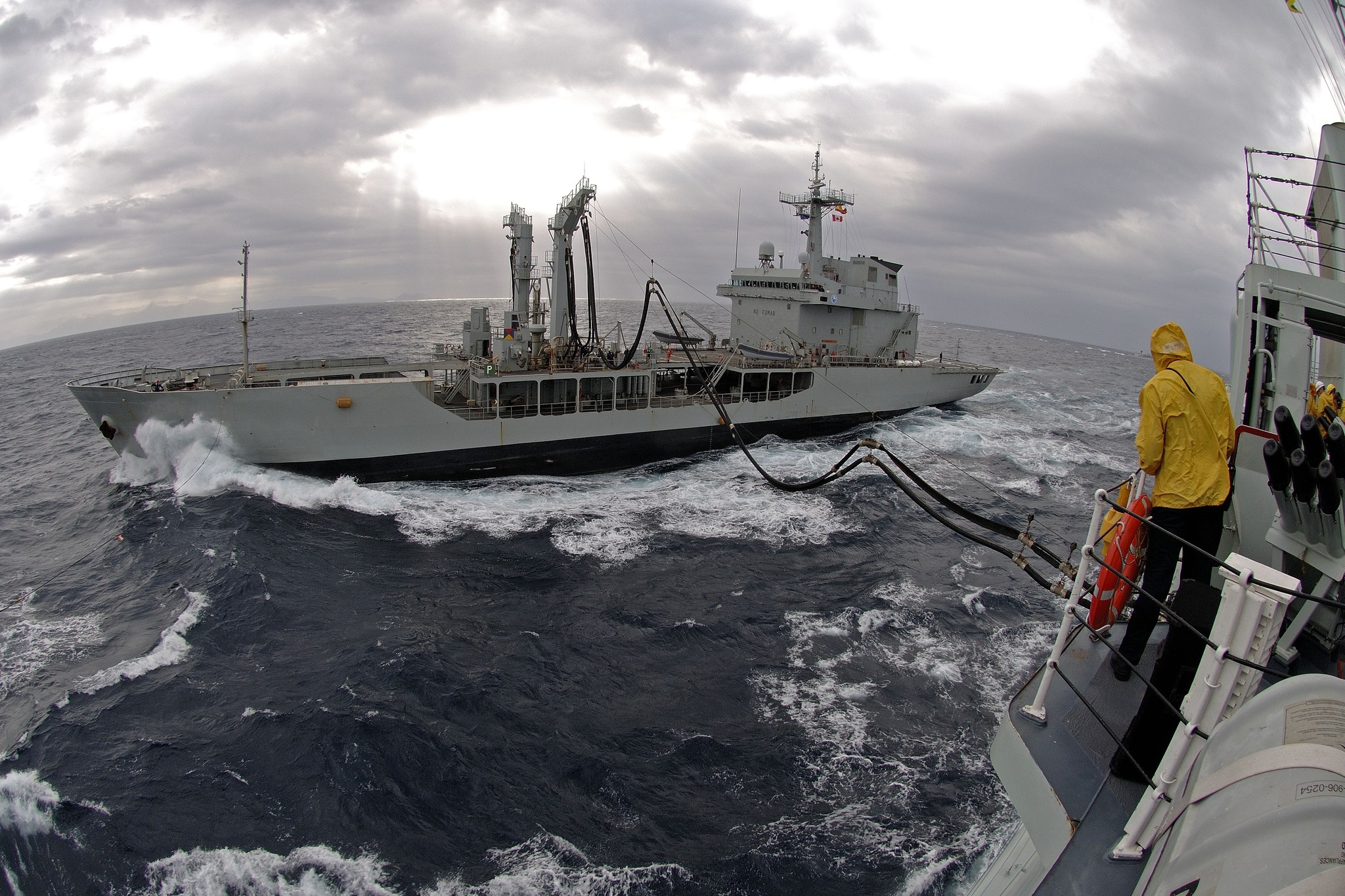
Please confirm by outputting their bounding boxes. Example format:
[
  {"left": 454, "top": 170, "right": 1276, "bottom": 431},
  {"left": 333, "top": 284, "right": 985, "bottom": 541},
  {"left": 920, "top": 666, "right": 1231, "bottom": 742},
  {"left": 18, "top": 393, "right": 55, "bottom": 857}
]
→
[
  {"left": 64, "top": 142, "right": 1005, "bottom": 483},
  {"left": 737, "top": 343, "right": 796, "bottom": 362},
  {"left": 653, "top": 329, "right": 705, "bottom": 346}
]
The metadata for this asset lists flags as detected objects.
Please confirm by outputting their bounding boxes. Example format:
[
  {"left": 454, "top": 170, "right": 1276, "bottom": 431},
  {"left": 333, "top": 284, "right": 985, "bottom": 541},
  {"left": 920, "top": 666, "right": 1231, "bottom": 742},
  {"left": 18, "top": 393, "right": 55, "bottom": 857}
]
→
[
  {"left": 835, "top": 202, "right": 847, "bottom": 214},
  {"left": 832, "top": 214, "right": 843, "bottom": 222},
  {"left": 1285, "top": 0, "right": 1303, "bottom": 14},
  {"left": 800, "top": 214, "right": 809, "bottom": 219}
]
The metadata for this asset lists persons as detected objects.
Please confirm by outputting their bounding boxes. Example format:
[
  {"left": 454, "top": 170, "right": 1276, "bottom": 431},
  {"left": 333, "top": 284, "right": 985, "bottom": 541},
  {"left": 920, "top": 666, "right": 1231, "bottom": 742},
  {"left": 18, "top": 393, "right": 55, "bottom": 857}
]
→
[
  {"left": 805, "top": 284, "right": 826, "bottom": 292},
  {"left": 1307, "top": 380, "right": 1345, "bottom": 437},
  {"left": 765, "top": 344, "right": 769, "bottom": 351},
  {"left": 834, "top": 274, "right": 839, "bottom": 282},
  {"left": 666, "top": 347, "right": 674, "bottom": 363},
  {"left": 808, "top": 348, "right": 844, "bottom": 366},
  {"left": 1109, "top": 325, "right": 1237, "bottom": 682},
  {"left": 155, "top": 377, "right": 160, "bottom": 392},
  {"left": 940, "top": 351, "right": 942, "bottom": 363}
]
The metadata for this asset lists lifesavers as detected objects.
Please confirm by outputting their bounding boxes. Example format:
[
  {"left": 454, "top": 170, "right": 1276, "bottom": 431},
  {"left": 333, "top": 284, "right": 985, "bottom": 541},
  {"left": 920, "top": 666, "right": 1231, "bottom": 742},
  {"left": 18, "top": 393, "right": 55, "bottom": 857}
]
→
[
  {"left": 719, "top": 355, "right": 723, "bottom": 361},
  {"left": 1088, "top": 494, "right": 1153, "bottom": 638}
]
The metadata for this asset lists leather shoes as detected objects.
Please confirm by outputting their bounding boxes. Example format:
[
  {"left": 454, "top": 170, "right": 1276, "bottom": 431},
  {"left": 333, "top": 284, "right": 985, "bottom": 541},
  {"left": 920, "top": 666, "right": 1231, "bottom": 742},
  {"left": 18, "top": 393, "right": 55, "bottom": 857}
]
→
[{"left": 1108, "top": 652, "right": 1131, "bottom": 682}]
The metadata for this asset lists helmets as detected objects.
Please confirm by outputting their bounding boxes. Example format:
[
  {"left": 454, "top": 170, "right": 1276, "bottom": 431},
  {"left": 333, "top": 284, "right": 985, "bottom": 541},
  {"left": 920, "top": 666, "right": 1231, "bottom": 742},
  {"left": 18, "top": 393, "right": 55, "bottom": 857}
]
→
[
  {"left": 1326, "top": 384, "right": 1336, "bottom": 395},
  {"left": 1315, "top": 381, "right": 1325, "bottom": 393}
]
